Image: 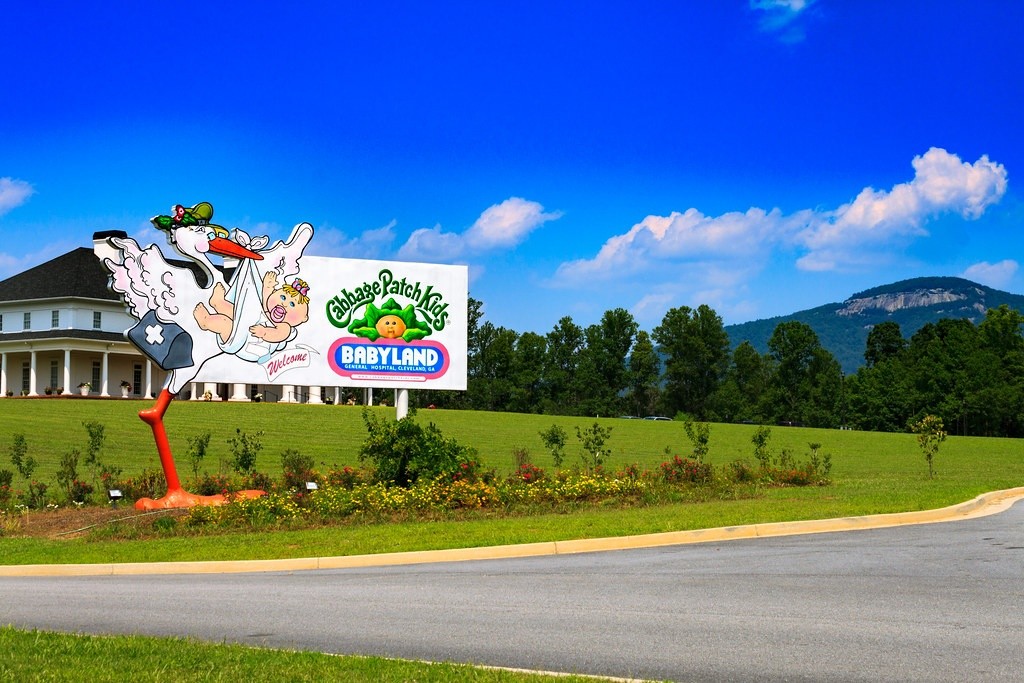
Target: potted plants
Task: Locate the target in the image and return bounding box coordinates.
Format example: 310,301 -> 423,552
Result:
45,386 -> 63,395
119,380 -> 132,398
76,382 -> 93,396
6,390 -> 29,397
